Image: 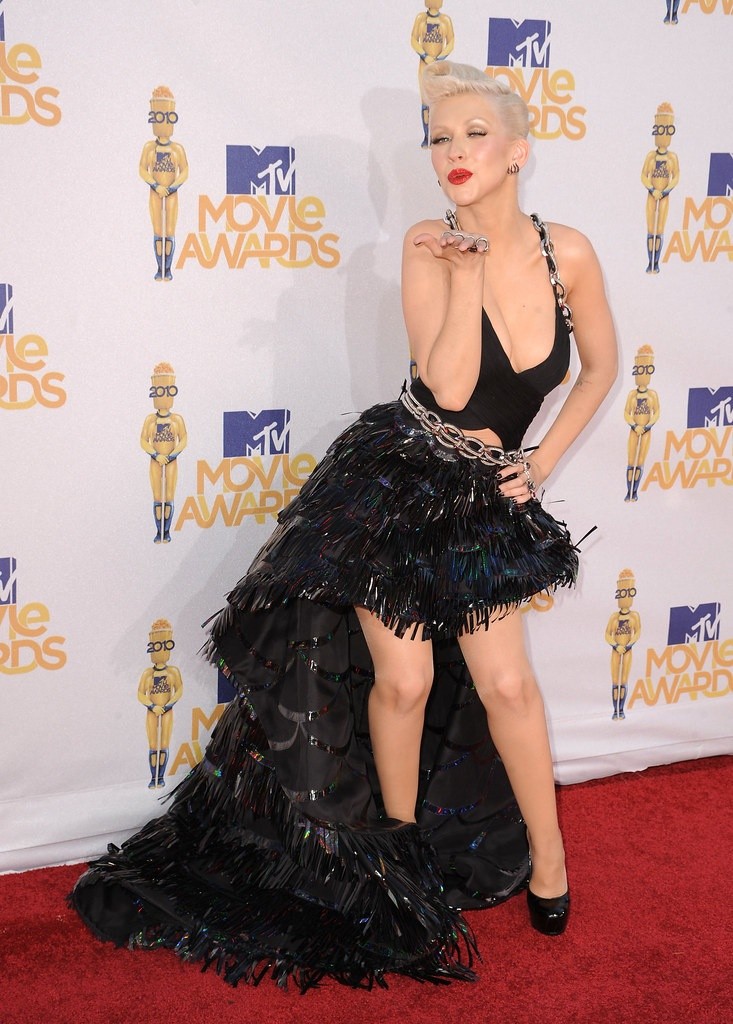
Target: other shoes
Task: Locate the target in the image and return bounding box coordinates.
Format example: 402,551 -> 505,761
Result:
526,846 -> 569,935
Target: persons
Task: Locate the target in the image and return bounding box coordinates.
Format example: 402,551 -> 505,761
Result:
331,60 -> 623,938
